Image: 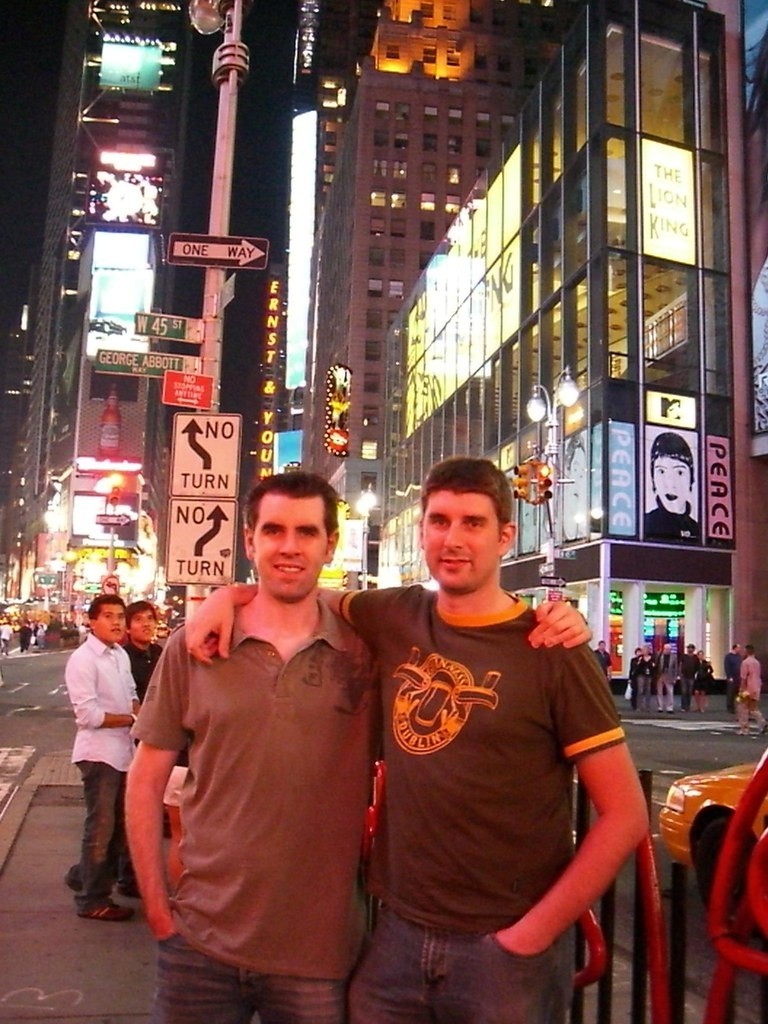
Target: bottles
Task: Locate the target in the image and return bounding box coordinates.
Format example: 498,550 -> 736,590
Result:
98,384 -> 124,458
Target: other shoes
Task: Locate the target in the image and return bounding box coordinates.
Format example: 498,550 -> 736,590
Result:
700,707 -> 704,713
666,707 -> 674,713
657,707 -> 663,712
681,708 -> 685,713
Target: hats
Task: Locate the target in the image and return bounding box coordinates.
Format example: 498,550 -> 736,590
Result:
745,644 -> 754,649
687,645 -> 695,648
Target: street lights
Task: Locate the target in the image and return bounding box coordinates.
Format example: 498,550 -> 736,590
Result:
353,484 -> 376,591
187,0 -> 248,633
525,364 -> 578,598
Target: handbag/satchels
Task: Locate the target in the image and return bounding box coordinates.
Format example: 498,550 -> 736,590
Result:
624,683 -> 633,701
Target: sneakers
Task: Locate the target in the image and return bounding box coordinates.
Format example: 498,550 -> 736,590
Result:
735,728 -> 748,736
117,881 -> 141,898
759,718 -> 768,734
76,897 -> 136,921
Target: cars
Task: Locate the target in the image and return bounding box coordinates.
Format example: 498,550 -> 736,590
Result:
1,616 -> 21,632
158,624 -> 170,638
657,754 -> 768,906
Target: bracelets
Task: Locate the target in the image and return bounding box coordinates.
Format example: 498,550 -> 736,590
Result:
129,713 -> 137,722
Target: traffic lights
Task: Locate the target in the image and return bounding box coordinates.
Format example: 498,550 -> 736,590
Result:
133,312 -> 205,344
538,463 -> 552,503
513,463 -> 529,503
108,476 -> 122,508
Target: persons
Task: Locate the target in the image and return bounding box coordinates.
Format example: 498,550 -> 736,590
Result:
63,594 -> 144,922
186,455 -> 649,1023
694,651 -> 714,712
731,643 -> 768,737
678,644 -> 701,713
631,646 -> 656,714
723,644 -> 742,714
629,648 -> 642,712
0,619 -> 91,656
120,473 -> 591,1023
121,600 -> 165,901
653,644 -> 679,713
592,641 -> 612,684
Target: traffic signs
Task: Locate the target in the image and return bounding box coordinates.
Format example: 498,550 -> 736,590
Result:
96,514 -> 131,526
164,410 -> 241,592
166,232 -> 270,271
91,347 -> 201,385
538,563 -> 556,576
541,578 -> 566,587
